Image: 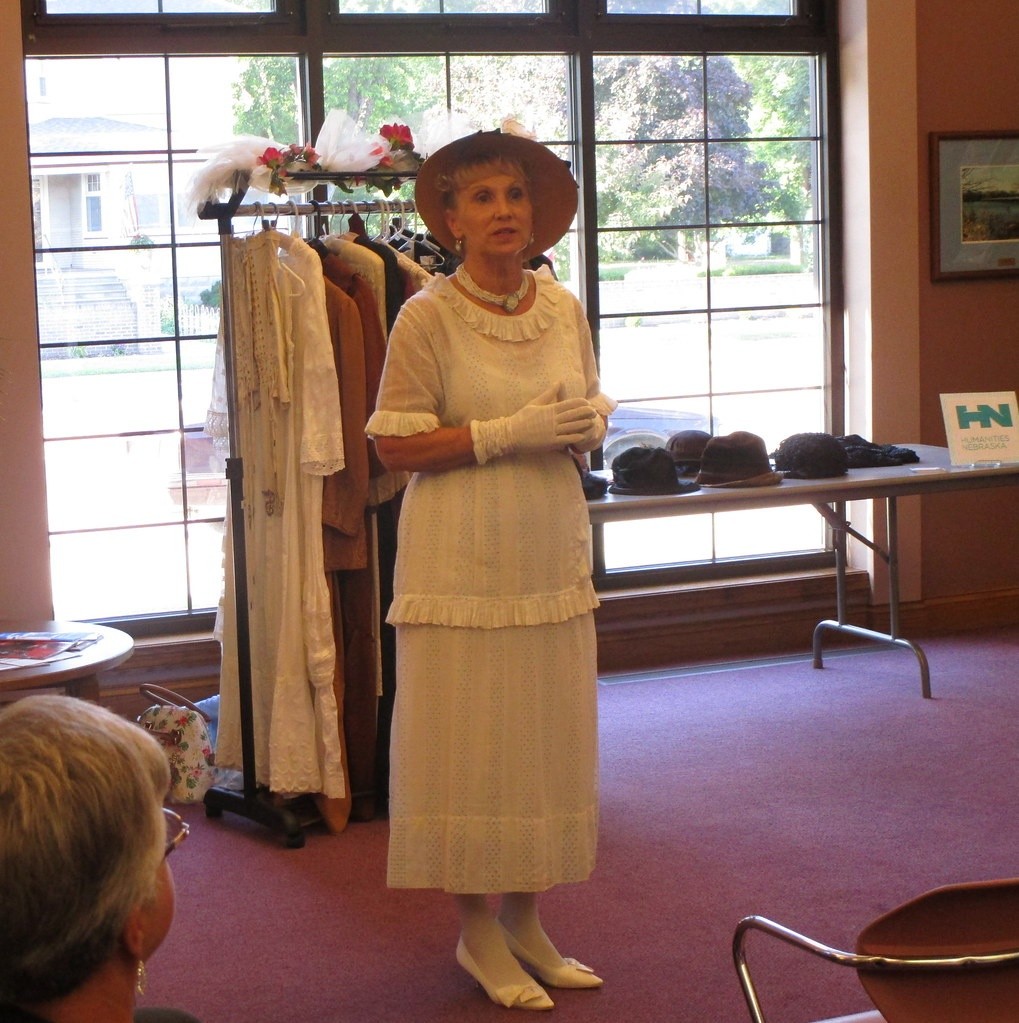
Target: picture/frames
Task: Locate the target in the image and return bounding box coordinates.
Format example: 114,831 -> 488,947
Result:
928,128 -> 1019,281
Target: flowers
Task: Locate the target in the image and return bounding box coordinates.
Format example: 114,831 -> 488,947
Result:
334,124 -> 425,197
259,142 -> 322,196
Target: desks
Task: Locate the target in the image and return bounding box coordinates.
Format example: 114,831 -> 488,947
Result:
581,443 -> 1019,696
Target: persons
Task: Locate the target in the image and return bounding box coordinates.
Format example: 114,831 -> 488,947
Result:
363,130 -> 616,1013
0,692 -> 197,1023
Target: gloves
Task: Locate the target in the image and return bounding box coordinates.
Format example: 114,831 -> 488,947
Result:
470,381 -> 597,465
557,384 -> 607,453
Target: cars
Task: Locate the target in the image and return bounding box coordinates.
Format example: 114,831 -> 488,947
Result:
167,399 -> 711,518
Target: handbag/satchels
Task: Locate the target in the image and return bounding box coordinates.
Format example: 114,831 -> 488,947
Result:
135,682 -> 216,805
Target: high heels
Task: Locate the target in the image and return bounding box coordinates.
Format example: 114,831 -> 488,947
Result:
454,933 -> 554,1011
494,914 -> 604,988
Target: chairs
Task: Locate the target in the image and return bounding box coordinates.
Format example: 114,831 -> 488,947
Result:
732,877 -> 1019,1022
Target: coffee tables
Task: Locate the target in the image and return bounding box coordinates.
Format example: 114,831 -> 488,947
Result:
0,619 -> 133,703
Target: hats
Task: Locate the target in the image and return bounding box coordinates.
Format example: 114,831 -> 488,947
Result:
664,430 -> 716,477
775,432 -> 848,478
569,449 -> 609,501
694,430 -> 784,487
609,445 -> 700,495
415,128 -> 580,261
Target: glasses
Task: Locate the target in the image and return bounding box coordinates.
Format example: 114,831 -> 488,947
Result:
160,806 -> 190,864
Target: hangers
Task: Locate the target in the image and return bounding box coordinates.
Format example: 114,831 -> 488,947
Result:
236,201 -> 446,300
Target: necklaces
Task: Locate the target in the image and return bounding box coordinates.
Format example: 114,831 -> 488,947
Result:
456,262 -> 530,313
